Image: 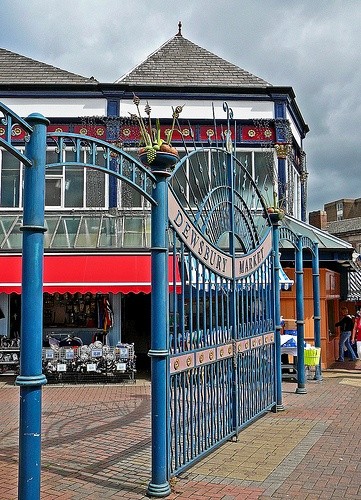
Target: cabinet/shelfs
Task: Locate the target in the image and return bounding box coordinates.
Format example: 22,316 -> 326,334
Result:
0,334 -> 138,385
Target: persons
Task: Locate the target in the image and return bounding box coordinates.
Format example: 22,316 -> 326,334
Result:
335,305 -> 358,362
351,306 -> 361,361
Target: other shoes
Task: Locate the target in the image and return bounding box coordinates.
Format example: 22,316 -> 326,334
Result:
349,360 -> 353,361
335,360 -> 342,362
